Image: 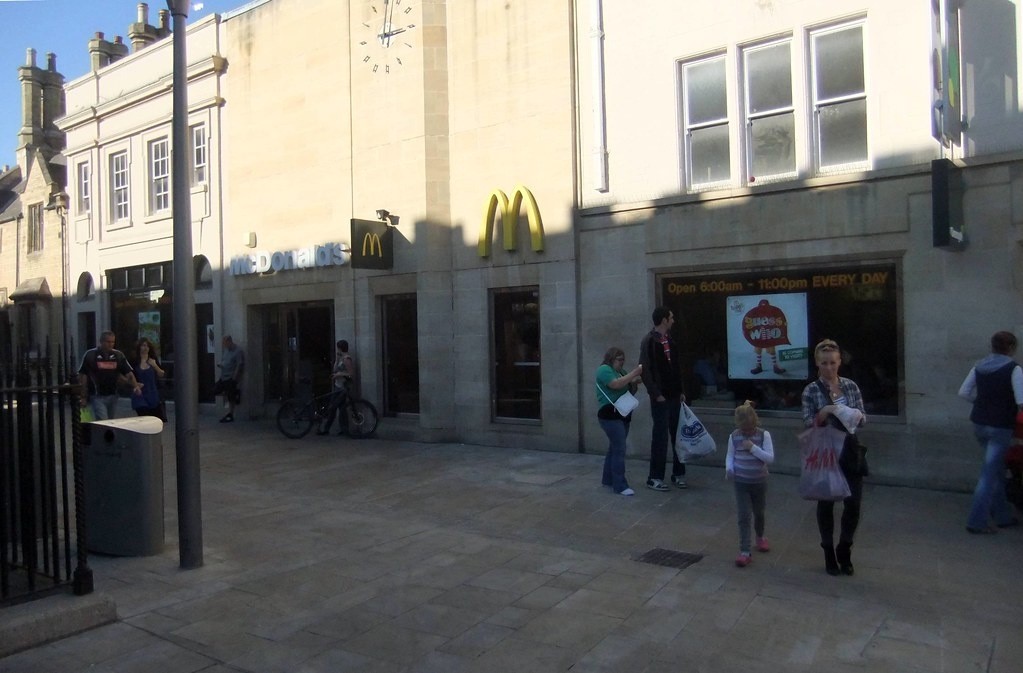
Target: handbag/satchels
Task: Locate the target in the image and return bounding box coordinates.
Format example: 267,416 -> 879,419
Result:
858,445 -> 869,477
155,404 -> 168,422
796,413 -> 852,502
80,405 -> 95,422
674,401 -> 717,464
613,391 -> 639,417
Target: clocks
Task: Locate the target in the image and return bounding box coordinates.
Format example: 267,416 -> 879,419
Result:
358,0 -> 416,73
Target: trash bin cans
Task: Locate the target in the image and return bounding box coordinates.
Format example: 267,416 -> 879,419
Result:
82,415 -> 166,557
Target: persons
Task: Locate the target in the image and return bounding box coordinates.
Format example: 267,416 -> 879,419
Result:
726,401 -> 773,565
959,332 -> 1023,533
78,331 -> 141,420
120,338 -> 165,416
802,340 -> 867,576
639,306 -> 688,490
318,340 -> 353,435
751,299 -> 785,374
213,336 -> 244,422
595,348 -> 643,496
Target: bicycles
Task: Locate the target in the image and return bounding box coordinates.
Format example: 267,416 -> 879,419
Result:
275,377 -> 380,440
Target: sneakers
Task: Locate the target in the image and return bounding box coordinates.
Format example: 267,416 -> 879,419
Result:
735,554 -> 751,566
671,475 -> 688,488
647,479 -> 669,490
755,536 -> 769,551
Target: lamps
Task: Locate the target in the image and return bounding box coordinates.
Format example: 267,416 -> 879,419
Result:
375,209 -> 388,222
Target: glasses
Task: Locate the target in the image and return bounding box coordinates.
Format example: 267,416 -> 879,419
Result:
615,358 -> 625,361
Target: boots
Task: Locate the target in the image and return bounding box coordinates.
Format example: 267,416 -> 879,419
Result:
836,544 -> 854,575
820,542 -> 839,577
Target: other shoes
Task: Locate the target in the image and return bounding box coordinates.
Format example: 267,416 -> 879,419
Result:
620,488 -> 635,495
966,525 -> 998,534
235,389 -> 242,405
219,413 -> 233,423
336,431 -> 344,435
318,431 -> 330,436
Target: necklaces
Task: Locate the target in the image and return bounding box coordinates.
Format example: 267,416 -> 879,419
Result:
831,390 -> 841,397
